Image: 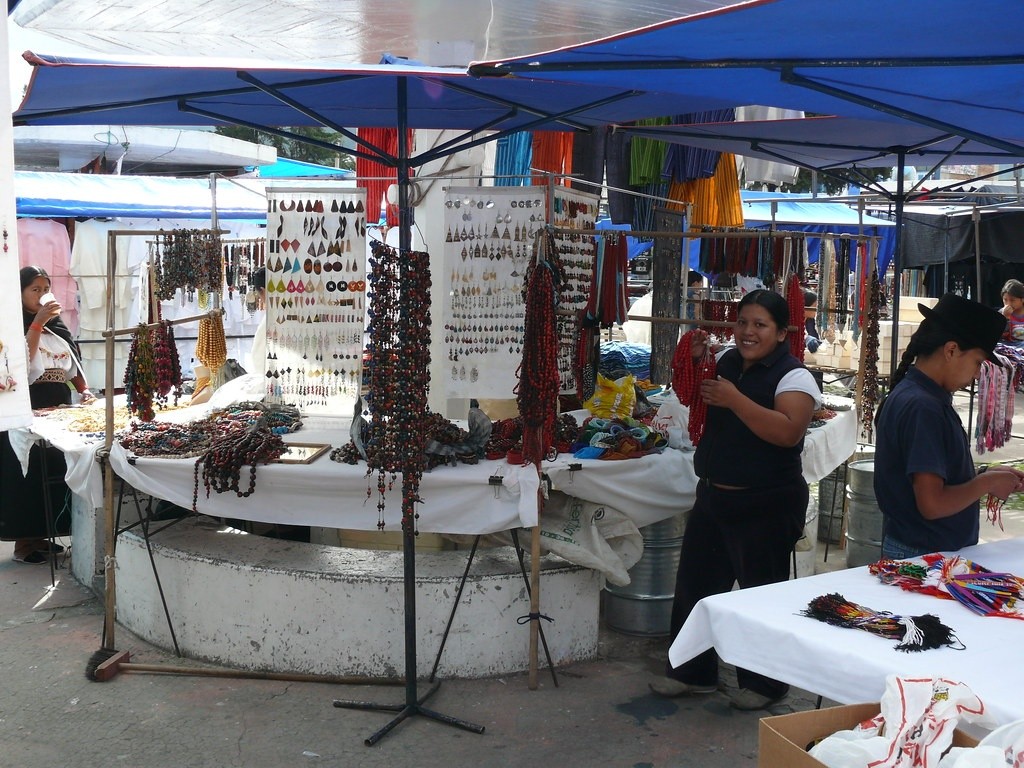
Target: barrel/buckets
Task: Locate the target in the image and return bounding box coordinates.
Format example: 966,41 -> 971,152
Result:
604,511 -> 687,635
845,459 -> 884,569
818,443 -> 875,544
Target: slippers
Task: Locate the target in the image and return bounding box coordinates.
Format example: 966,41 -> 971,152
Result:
11,552 -> 47,565
35,542 -> 63,553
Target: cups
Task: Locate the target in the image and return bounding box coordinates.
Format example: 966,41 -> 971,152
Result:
40,293 -> 61,313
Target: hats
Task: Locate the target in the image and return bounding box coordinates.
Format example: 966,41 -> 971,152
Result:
916,293 -> 1008,367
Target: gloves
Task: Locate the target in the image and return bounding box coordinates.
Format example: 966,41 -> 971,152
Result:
805,335 -> 823,353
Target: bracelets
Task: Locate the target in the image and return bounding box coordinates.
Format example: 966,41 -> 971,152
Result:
28,322 -> 43,333
485,412 -> 667,463
978,465 -> 988,474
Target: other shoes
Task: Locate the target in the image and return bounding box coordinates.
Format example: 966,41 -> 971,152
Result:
649,676 -> 719,696
731,687 -> 790,711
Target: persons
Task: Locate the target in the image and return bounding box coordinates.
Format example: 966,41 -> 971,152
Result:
649,289 -> 821,710
997,279 -> 1024,333
1,266 -> 94,563
874,292 -> 1024,562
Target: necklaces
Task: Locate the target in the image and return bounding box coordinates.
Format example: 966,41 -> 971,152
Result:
580,227 -> 879,449
69,228 -> 571,536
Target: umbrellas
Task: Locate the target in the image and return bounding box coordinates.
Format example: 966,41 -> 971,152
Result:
12,0 -> 1024,370
